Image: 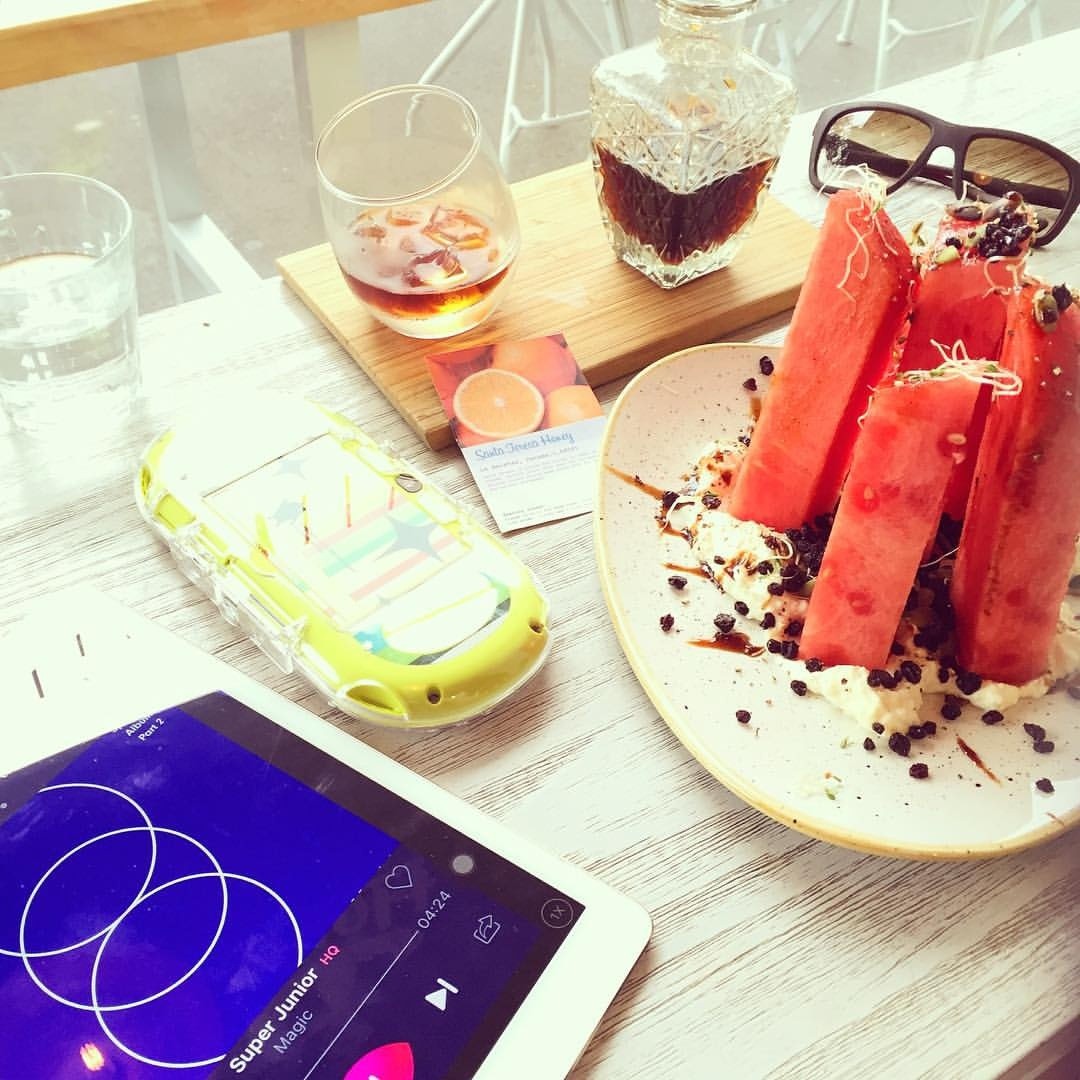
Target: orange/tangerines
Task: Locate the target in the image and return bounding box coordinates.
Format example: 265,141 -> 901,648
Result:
423,336 -> 602,448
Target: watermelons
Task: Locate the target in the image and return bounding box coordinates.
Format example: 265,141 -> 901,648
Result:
725,181 -> 1080,689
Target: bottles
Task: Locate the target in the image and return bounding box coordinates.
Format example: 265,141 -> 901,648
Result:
585,0 -> 798,292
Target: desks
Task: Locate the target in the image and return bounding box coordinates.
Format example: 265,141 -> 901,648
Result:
0,28 -> 1080,1080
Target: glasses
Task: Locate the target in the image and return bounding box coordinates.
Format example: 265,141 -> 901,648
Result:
806,98 -> 1076,249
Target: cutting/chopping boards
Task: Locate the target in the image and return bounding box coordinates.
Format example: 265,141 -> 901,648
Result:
272,153 -> 861,452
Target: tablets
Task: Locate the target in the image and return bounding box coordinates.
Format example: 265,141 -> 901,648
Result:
0,591 -> 653,1080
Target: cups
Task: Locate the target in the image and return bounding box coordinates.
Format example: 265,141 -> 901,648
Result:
0,169 -> 141,438
315,83 -> 518,339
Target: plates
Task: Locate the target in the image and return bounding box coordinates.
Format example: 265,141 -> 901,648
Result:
596,333 -> 1080,856
